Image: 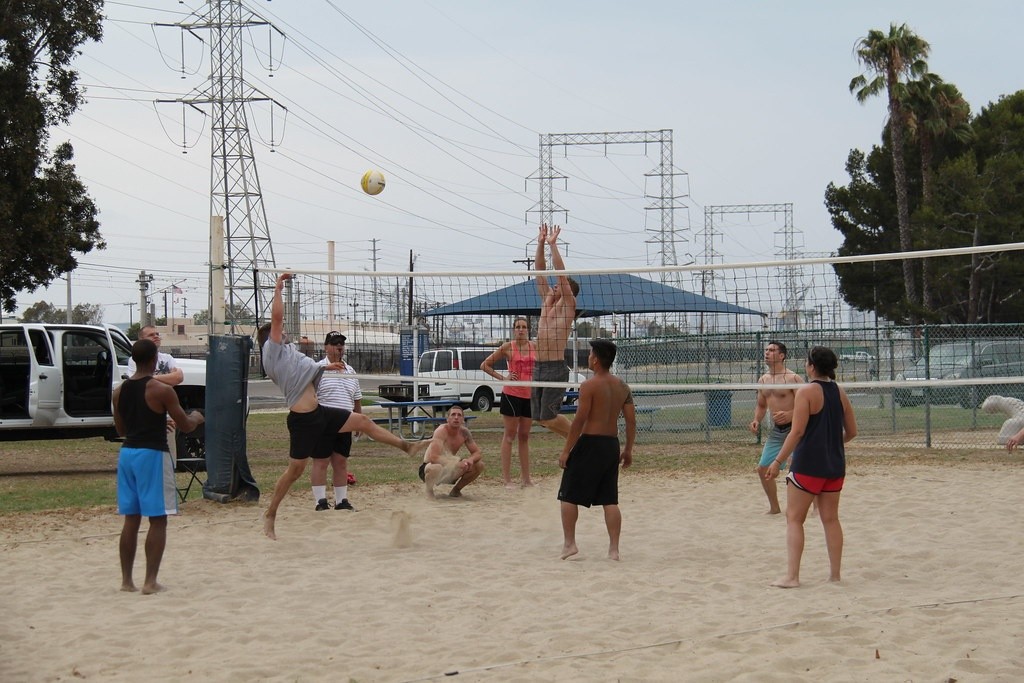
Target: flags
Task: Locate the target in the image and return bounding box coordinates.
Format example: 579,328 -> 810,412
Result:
173,286 -> 182,294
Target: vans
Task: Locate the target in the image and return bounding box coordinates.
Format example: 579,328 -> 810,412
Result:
417,347 -> 587,412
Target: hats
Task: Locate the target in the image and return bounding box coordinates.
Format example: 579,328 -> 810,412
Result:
325,330 -> 347,345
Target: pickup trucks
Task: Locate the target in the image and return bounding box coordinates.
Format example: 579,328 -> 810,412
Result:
839,351 -> 876,364
0,322 -> 251,440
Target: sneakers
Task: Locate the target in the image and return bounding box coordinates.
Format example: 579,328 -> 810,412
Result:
315,497 -> 332,511
335,498 -> 357,512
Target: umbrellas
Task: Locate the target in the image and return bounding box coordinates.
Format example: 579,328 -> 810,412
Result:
414,273 -> 768,392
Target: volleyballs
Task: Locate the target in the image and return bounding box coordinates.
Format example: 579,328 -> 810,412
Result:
361,169 -> 385,195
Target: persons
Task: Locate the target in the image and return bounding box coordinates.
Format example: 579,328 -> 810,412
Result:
112,339 -> 205,594
481,317 -> 536,486
128,325 -> 183,514
258,271 -> 433,540
1006,427 -> 1024,452
310,331 -> 356,511
869,357 -> 876,380
749,342 -> 819,520
530,224 -> 580,437
420,407 -> 485,498
557,340 -> 636,562
764,346 -> 857,587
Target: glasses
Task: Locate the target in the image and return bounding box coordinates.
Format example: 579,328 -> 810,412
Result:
328,340 -> 345,346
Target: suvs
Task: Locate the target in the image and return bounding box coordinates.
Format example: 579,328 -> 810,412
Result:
892,337 -> 1024,407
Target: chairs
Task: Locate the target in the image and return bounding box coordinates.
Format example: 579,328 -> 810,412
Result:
174,429 -> 205,502
0,366 -> 27,411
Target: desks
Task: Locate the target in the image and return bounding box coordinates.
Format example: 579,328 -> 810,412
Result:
372,400 -> 460,441
565,391 -> 579,398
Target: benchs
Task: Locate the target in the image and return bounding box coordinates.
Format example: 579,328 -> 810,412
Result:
371,416 -> 477,422
559,406 -> 662,415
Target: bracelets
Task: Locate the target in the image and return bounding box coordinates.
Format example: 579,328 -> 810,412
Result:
775,458 -> 782,465
